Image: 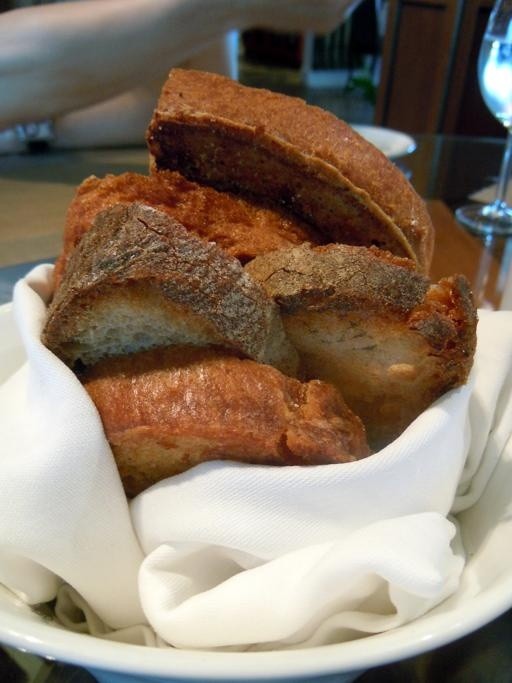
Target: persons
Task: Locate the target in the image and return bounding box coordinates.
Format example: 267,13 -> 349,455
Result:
0,0 -> 362,157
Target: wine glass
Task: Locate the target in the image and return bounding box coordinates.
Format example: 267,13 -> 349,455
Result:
457,0 -> 512,243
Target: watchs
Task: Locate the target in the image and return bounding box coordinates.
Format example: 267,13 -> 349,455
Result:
15,117 -> 57,155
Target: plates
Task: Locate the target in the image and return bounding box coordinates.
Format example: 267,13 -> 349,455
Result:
0,292 -> 509,682
316,122 -> 418,167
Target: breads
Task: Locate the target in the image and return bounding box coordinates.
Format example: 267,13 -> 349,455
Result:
41,65 -> 480,496
